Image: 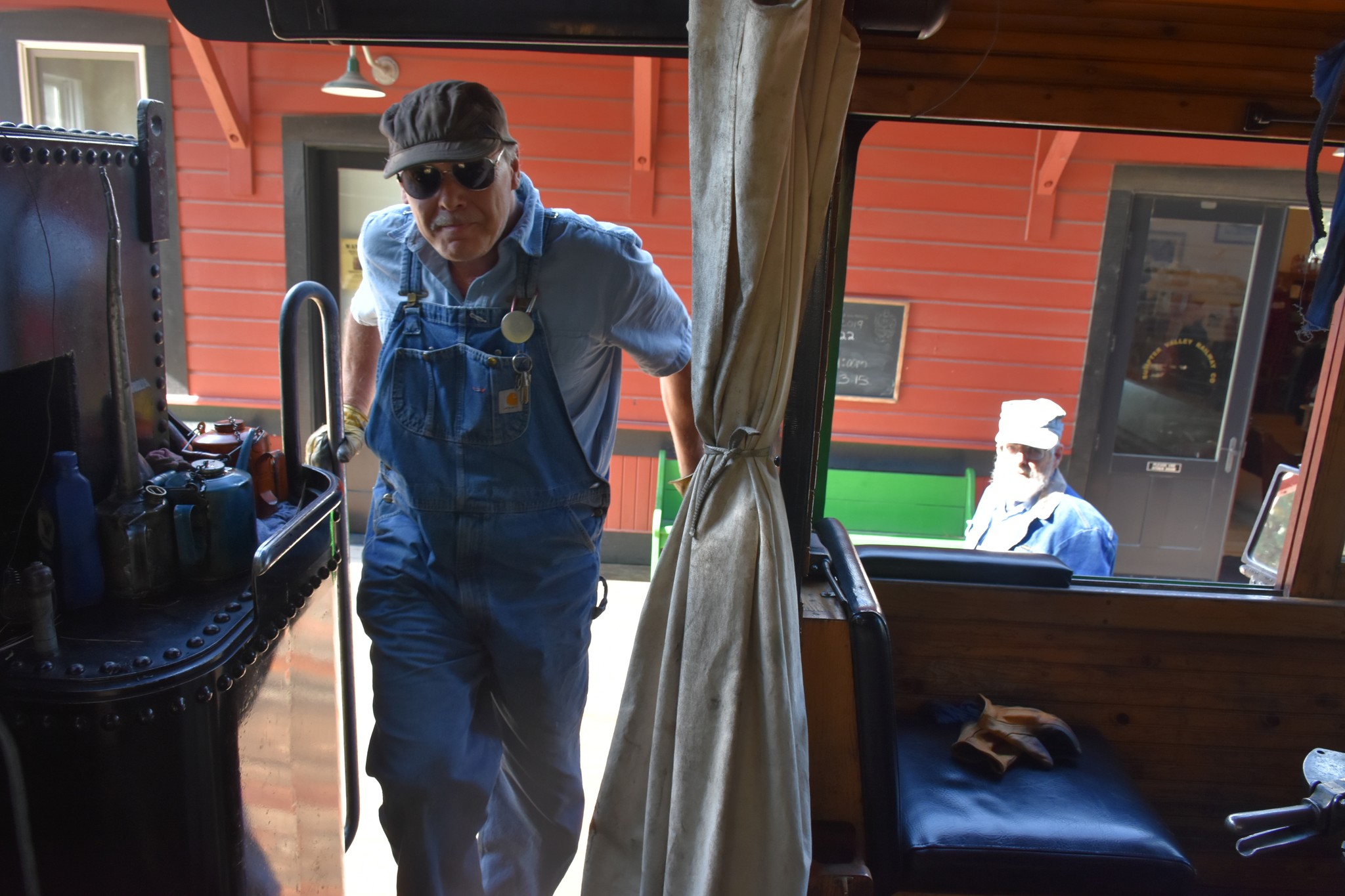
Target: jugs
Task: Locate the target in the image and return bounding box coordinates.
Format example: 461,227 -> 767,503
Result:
106,418 -> 271,607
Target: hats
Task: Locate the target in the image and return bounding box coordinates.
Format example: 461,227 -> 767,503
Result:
995,398 -> 1067,449
378,80 -> 518,178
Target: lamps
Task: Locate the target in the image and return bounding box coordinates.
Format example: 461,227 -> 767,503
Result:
321,44 -> 399,97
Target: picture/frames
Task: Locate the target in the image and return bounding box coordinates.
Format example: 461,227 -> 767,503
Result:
1144,230 -> 1186,268
1214,223 -> 1259,244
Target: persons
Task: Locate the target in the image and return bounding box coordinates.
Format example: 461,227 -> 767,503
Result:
305,80 -> 704,896
965,398 -> 1118,576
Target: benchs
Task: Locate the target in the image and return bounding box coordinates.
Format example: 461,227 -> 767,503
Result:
649,450 -> 976,578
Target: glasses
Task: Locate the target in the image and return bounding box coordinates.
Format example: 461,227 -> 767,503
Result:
396,149 -> 505,199
996,445 -> 1050,460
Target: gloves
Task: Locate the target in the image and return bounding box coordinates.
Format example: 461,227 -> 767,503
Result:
306,404 -> 367,473
977,691 -> 1081,767
953,724 -> 1019,773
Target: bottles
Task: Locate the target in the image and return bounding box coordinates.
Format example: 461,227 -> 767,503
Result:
46,452 -> 105,612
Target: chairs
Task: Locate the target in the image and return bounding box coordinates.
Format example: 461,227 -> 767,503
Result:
810,513 -> 1195,895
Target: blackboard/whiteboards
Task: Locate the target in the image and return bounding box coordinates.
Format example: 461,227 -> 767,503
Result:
835,295 -> 911,405
